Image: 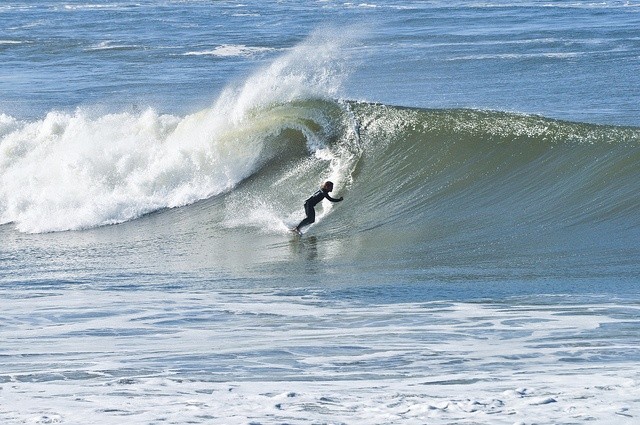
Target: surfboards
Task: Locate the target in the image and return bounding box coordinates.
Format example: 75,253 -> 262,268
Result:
283,222 -> 303,236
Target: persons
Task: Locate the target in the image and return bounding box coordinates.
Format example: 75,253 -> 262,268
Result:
292,181 -> 344,232
